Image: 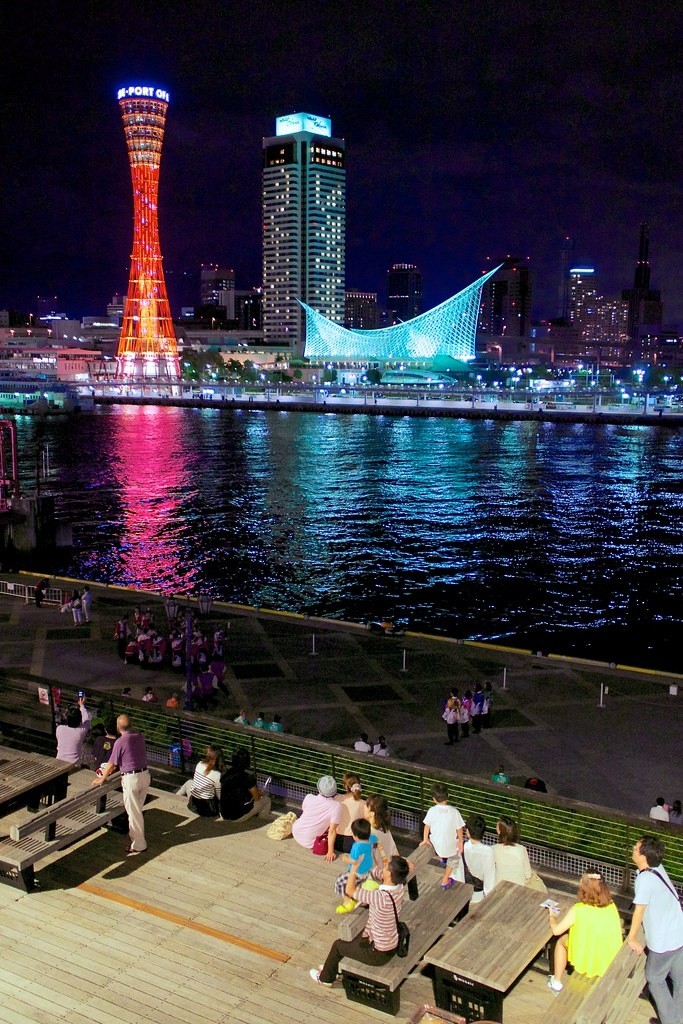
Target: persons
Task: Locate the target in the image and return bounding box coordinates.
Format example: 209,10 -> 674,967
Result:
354,733 -> 390,756
113,606 -> 224,672
335,795 -> 399,914
176,745 -> 271,822
291,775 -> 342,862
548,868 -> 624,997
310,853 -> 409,987
121,687 -> 180,709
649,797 -> 683,825
56,695 -> 119,771
419,784 -> 532,902
71,586 -> 93,626
92,715 -> 151,852
524,778 -> 547,794
627,836 -> 683,1024
492,765 -> 510,784
34,578 -> 50,608
334,773 -> 365,853
442,682 -> 492,745
233,709 -> 283,732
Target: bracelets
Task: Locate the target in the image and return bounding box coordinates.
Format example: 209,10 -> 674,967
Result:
550,915 -> 556,919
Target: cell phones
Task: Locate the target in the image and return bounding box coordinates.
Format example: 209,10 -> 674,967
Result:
78,690 -> 83,700
547,903 -> 558,913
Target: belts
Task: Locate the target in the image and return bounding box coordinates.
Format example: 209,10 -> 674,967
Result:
121,767 -> 148,776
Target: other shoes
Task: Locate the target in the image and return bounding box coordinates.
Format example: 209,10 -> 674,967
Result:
649,1017 -> 662,1024
125,845 -> 147,852
441,878 -> 453,890
547,975 -> 564,996
335,899 -> 355,914
441,858 -> 447,867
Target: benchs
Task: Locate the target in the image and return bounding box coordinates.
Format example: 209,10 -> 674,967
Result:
337,837 -> 474,1016
538,920 -> 648,1024
0,770 -> 127,895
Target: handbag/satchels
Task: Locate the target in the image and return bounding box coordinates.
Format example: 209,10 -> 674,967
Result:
73,599 -> 81,608
461,841 -> 484,892
397,922 -> 410,957
267,812 -> 297,840
42,589 -> 47,594
313,826 -> 329,855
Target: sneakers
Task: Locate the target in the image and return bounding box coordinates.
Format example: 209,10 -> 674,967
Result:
319,964 -> 343,981
309,969 -> 333,987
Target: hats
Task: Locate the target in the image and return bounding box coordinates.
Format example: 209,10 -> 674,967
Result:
317,775 -> 337,797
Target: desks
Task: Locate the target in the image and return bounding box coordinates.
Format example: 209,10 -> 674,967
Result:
0,752 -> 75,820
424,879 -> 579,1024
